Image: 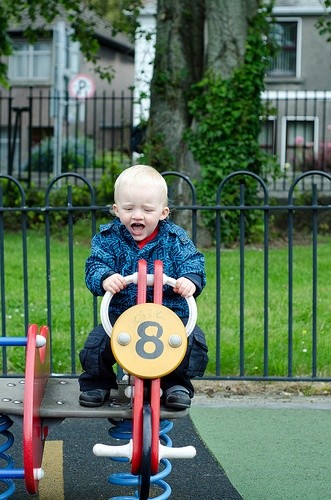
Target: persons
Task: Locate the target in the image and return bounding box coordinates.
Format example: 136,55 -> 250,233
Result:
78,164 -> 209,412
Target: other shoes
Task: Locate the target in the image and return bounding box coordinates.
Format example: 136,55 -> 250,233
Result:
79,389 -> 111,406
166,386 -> 191,408
